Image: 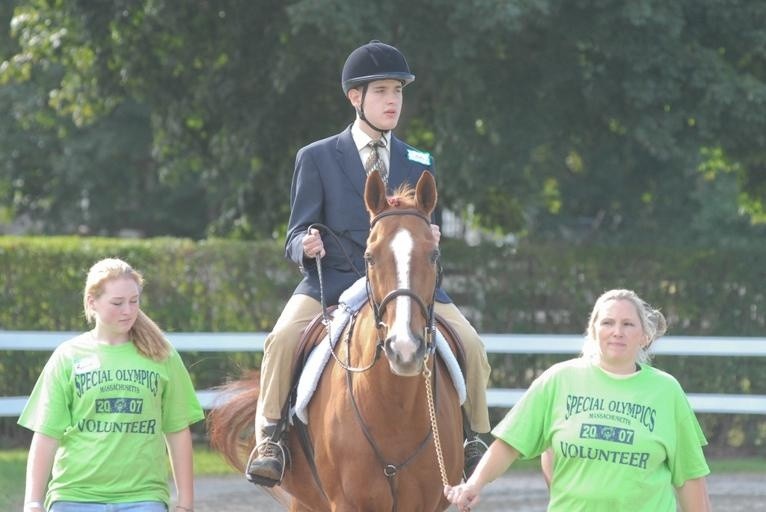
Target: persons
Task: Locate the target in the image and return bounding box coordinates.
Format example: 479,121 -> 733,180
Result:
248,39 -> 492,481
16,257 -> 205,512
442,288 -> 711,512
541,310 -> 667,488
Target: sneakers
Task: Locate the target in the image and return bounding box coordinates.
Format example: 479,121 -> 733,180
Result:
464,432 -> 489,479
248,424 -> 292,482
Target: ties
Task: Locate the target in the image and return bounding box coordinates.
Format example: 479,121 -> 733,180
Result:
365,138 -> 388,182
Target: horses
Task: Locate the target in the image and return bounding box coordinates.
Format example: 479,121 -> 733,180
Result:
205,168 -> 465,512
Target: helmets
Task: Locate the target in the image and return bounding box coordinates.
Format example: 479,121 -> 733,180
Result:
341,39 -> 416,97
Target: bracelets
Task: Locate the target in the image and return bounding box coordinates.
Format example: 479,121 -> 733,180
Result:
176,505 -> 194,512
